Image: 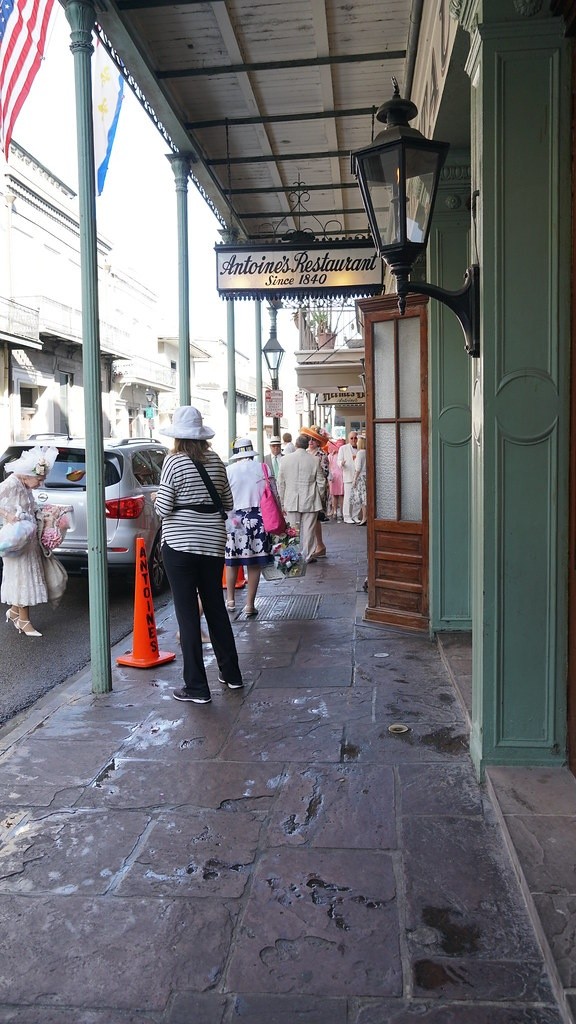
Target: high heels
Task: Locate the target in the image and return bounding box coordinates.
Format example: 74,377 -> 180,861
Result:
16,617 -> 42,636
313,546 -> 327,558
5,608 -> 19,630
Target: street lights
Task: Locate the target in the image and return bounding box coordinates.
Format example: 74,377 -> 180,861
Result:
263,307 -> 285,455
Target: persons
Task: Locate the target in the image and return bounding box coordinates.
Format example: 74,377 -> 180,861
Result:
151,406 -> 244,704
0,445 -> 68,637
320,432 -> 367,526
264,425 -> 329,563
177,587 -> 212,643
223,438 -> 282,615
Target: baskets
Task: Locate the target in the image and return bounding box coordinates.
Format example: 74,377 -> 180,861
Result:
262,530 -> 307,582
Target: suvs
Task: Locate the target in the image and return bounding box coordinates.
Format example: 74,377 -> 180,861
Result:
0,432 -> 174,596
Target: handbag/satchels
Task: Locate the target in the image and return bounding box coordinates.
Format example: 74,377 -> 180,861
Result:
0,508 -> 38,557
261,462 -> 287,534
36,513 -> 68,610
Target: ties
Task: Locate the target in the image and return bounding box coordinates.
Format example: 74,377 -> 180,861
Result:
274,456 -> 279,481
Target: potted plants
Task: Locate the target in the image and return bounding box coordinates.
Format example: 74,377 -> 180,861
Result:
309,310 -> 337,349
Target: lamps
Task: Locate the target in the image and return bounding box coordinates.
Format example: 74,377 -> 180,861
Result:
348,79 -> 479,360
359,357 -> 365,391
337,385 -> 347,391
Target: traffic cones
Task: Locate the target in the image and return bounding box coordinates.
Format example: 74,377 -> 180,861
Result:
116,538 -> 177,668
221,562 -> 249,589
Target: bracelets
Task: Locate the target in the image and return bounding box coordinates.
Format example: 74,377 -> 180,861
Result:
5,511 -> 7,520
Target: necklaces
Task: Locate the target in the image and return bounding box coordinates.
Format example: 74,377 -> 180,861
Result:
22,477 -> 32,514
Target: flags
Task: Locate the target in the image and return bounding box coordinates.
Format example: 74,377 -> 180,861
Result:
96,22 -> 124,196
0,0 -> 55,162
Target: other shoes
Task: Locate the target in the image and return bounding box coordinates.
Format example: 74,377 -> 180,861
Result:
199,634 -> 212,642
327,509 -> 368,526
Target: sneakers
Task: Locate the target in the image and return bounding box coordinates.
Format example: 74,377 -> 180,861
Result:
172,688 -> 212,704
218,670 -> 244,689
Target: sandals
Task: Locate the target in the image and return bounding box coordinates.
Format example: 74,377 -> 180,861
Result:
246,605 -> 258,616
225,599 -> 236,612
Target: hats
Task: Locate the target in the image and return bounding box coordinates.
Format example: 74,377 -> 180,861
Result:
228,438 -> 260,459
268,436 -> 283,446
159,407 -> 215,439
301,425 -> 330,446
